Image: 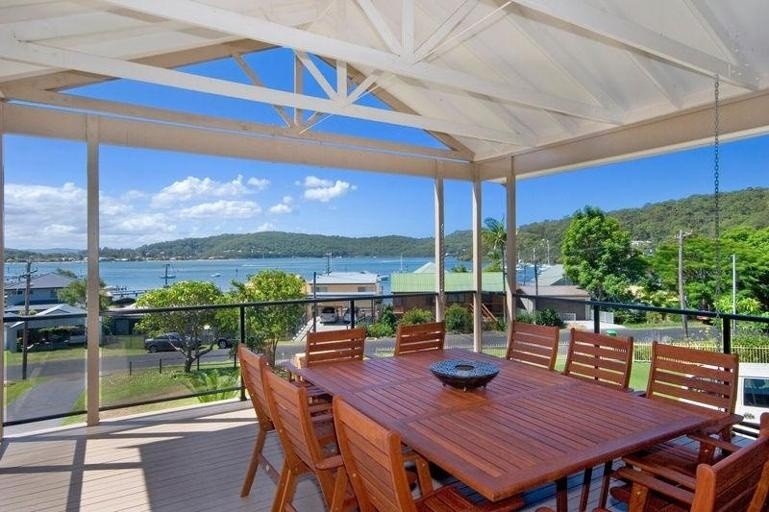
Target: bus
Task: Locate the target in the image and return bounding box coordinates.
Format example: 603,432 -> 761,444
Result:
69,325 -> 112,345
678,361 -> 769,426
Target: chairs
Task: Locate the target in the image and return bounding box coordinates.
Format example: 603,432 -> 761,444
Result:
262,362 -> 418,512
506,323 -> 559,371
395,322 -> 445,355
304,326 -> 365,367
564,328 -> 634,388
332,393 -> 524,512
237,343 -> 331,512
610,340 -> 744,502
591,412 -> 768,512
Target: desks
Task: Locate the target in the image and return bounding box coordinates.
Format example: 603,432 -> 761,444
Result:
295,347 -> 712,512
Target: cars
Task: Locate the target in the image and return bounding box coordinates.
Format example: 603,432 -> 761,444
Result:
219,336 -> 263,348
344,307 -> 365,322
144,332 -> 201,351
320,306 -> 338,324
